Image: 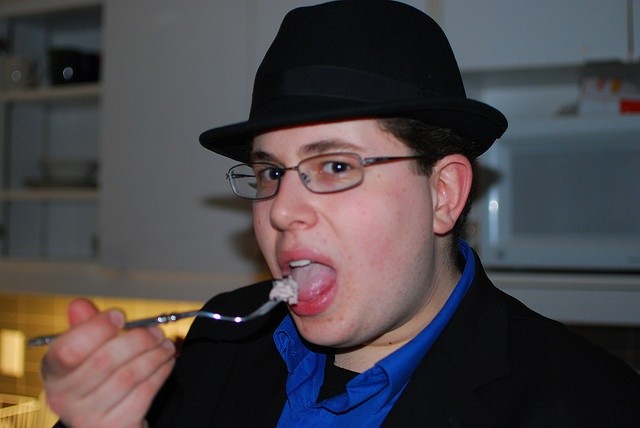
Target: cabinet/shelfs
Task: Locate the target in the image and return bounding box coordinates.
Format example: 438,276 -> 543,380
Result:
0,0 -> 104,266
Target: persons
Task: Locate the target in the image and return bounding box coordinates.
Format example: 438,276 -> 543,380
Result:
40,1 -> 640,428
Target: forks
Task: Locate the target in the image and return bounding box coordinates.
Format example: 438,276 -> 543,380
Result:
26,294 -> 287,347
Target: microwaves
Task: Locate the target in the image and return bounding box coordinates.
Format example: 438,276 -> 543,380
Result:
460,116 -> 640,273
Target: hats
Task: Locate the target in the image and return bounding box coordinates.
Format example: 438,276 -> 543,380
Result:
199,0 -> 508,167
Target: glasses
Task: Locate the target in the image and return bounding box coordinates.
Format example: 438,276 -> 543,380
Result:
225,152 -> 439,200
